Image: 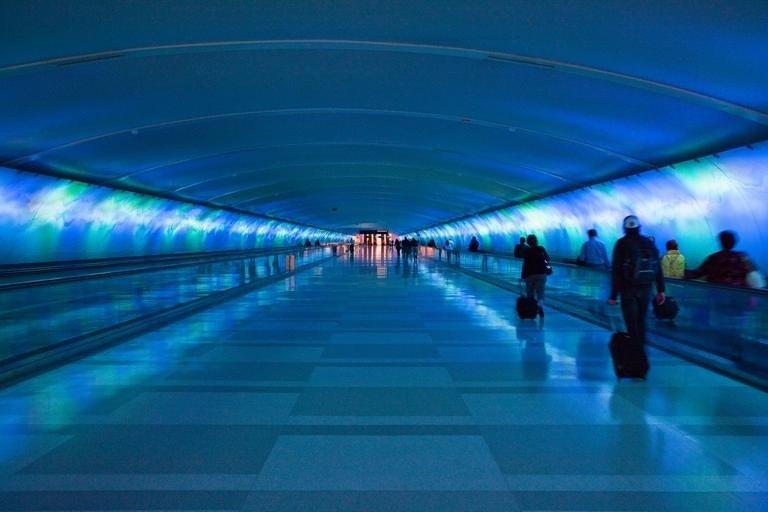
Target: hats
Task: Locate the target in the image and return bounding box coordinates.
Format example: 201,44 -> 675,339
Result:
623,215 -> 641,229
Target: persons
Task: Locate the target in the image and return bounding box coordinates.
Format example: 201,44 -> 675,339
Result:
395,237 -> 453,259
660,239 -> 686,280
578,230 -> 609,271
609,214 -> 666,338
514,238 -> 527,258
470,236 -> 479,252
686,231 -> 755,288
350,238 -> 354,253
522,235 -> 549,318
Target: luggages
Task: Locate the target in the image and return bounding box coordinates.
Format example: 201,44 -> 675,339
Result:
517,279 -> 538,319
652,292 -> 678,319
607,302 -> 648,379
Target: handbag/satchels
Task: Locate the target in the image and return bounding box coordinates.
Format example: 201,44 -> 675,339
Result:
544,259 -> 553,275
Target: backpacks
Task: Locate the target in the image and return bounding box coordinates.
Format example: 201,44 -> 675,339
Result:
625,238 -> 661,286
